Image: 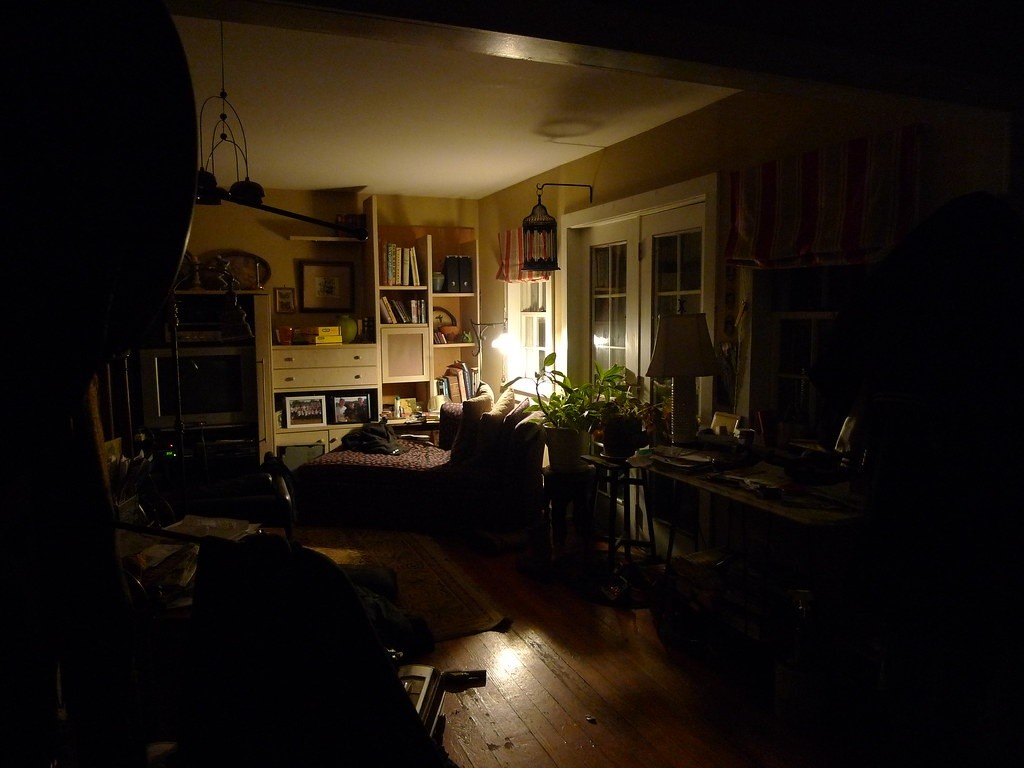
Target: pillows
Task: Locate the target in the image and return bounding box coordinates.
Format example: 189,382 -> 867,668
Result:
449,381 -> 495,459
471,386 -> 514,458
498,397 -> 530,455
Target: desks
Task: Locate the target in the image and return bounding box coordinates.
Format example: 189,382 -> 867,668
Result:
577,453 -> 659,584
629,442 -> 881,694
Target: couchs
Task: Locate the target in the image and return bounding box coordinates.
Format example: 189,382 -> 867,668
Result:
301,381 -> 548,533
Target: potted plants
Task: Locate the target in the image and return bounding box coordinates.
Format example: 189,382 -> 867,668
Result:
500,352 -> 672,457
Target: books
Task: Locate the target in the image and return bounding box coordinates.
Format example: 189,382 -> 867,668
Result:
434,359 -> 479,404
380,239 -> 421,287
380,295 -> 426,325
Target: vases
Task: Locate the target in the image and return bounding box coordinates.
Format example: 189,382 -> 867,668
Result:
432,272 -> 445,292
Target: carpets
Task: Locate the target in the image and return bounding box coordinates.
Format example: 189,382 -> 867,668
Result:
289,527 -> 509,641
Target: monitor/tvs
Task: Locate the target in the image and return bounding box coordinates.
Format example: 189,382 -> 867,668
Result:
139,346 -> 258,429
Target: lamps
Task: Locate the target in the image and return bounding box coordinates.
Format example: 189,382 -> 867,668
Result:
468,318 -> 518,381
647,300 -> 724,447
520,182 -> 592,272
194,19 -> 266,207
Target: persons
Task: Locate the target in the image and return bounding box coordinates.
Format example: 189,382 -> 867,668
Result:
290,400 -> 321,420
336,397 -> 369,423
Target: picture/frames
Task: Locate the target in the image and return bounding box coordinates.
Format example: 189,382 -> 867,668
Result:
285,396 -> 327,428
216,252 -> 271,290
331,395 -> 372,424
297,260 -> 355,313
275,287 -> 295,313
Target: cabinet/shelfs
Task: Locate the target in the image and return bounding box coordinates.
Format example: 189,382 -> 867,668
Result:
271,196 -> 483,471
